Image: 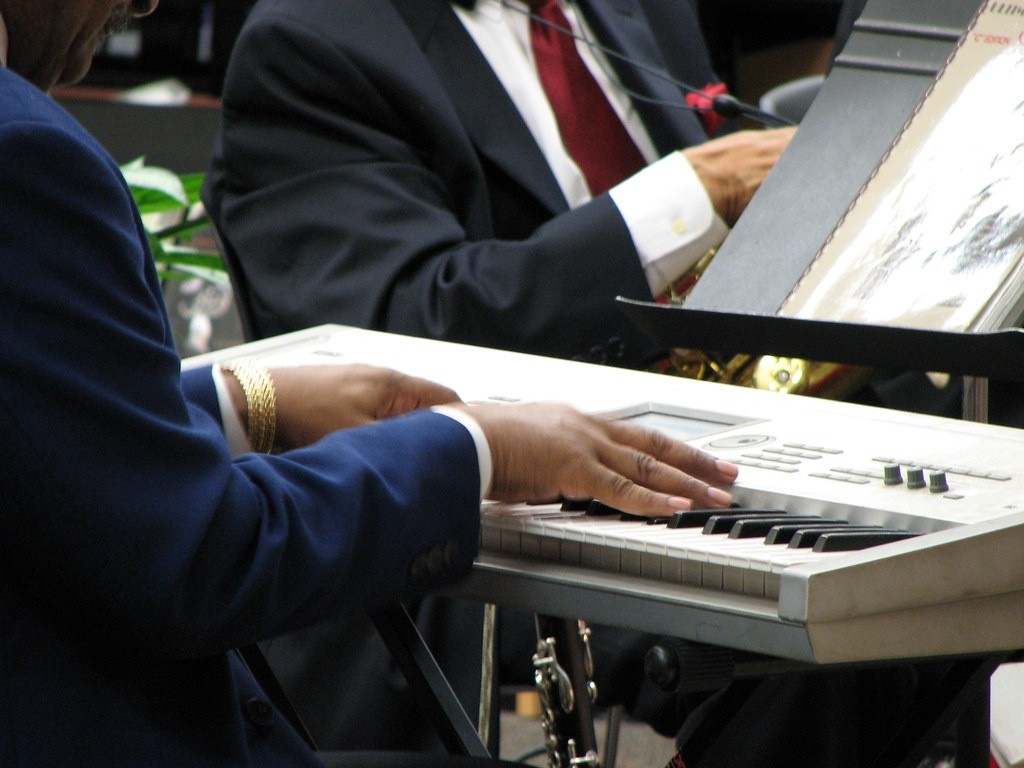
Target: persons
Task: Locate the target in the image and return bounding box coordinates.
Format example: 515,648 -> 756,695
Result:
206,2 -> 988,765
0,4 -> 743,768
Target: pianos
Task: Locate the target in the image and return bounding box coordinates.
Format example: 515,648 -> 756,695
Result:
179,320 -> 1024,670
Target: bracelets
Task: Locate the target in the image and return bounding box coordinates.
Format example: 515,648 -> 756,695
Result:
219,356 -> 278,456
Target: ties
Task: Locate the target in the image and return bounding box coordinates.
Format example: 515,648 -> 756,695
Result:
525,0 -> 701,306
